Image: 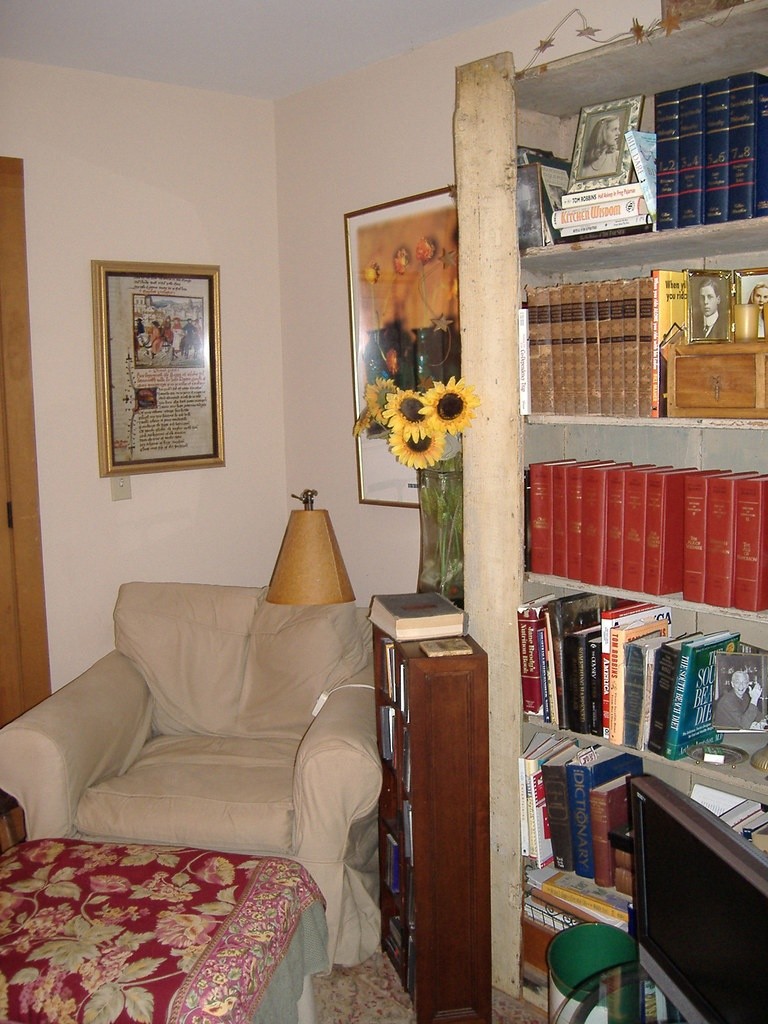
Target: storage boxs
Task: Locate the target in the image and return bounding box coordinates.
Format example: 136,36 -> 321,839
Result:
666,341 -> 768,418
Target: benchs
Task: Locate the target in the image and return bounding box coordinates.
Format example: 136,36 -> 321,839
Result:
0,838 -> 328,1024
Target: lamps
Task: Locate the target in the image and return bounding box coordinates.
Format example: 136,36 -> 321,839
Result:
266,489 -> 356,605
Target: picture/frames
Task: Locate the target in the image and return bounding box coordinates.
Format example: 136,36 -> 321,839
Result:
734,267 -> 768,341
685,270 -> 734,343
91,259 -> 226,478
565,93 -> 644,194
344,185 -> 462,508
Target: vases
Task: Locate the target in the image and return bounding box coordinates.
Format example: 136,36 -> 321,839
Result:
418,469 -> 464,610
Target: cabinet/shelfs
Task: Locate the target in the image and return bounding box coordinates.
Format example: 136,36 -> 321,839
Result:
372,625 -> 492,1024
455,0 -> 768,1024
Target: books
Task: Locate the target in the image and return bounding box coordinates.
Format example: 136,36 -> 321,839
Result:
518,72 -> 768,245
518,731 -> 768,933
381,638 -> 413,992
522,894 -> 582,934
524,458 -> 768,611
517,593 -> 742,762
368,592 -> 469,640
518,271 -> 688,418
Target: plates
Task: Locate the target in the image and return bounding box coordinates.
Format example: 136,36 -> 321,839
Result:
685,744 -> 748,766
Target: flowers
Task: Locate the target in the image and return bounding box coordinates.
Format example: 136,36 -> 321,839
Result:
353,376 -> 481,597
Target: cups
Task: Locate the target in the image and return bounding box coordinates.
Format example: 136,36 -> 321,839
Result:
734,304 -> 759,343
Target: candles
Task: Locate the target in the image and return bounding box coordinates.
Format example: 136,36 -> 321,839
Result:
733,303 -> 759,341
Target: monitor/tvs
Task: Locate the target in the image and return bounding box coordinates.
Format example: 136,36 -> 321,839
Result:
626,773 -> 768,1024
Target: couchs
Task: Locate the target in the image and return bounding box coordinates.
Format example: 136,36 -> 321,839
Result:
0,582 -> 383,976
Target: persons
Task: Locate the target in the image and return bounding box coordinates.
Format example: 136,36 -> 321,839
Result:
581,116 -> 619,174
694,279 -> 724,338
715,671 -> 763,729
748,284 -> 768,337
